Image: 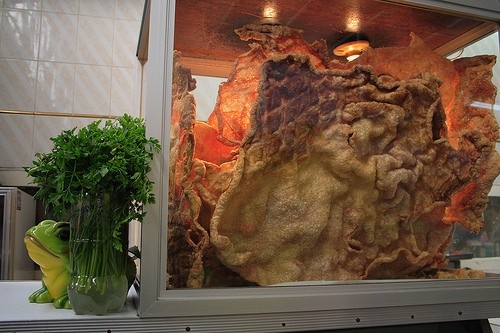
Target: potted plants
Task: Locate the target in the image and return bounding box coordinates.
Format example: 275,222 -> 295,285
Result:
22,113 -> 163,316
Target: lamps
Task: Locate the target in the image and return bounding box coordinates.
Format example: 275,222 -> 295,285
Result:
333,40 -> 370,61
